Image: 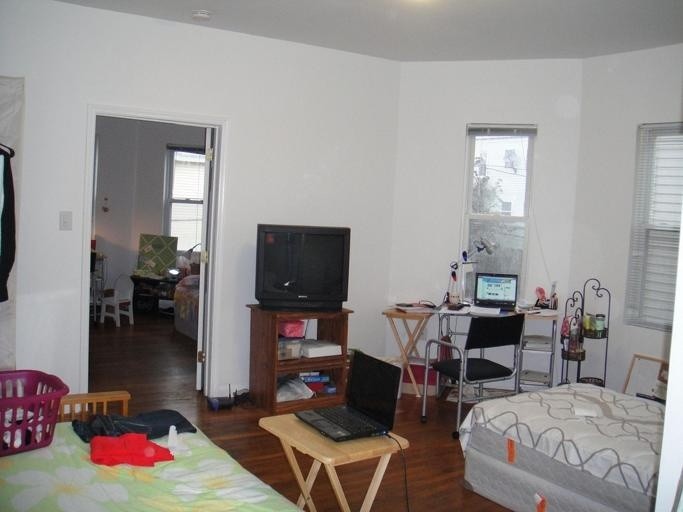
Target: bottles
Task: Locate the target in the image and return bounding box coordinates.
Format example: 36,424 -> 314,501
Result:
165,424 -> 179,454
448,271 -> 460,304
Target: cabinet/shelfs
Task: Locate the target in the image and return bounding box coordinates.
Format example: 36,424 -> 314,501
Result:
246,303 -> 354,415
557,278 -> 612,388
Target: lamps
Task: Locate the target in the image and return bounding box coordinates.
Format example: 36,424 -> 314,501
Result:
443,237 -> 497,305
183,243 -> 201,259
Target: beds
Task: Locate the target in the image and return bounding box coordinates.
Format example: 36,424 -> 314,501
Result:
0,391 -> 306,512
172,275 -> 199,343
459,382 -> 665,512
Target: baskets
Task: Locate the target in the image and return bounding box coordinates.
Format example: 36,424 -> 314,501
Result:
0,367 -> 71,459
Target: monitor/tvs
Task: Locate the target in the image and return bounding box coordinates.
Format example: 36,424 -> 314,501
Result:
255,224 -> 350,309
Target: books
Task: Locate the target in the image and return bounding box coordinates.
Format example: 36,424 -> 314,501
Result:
300,374 -> 329,382
298,371 -> 320,376
394,304 -> 500,316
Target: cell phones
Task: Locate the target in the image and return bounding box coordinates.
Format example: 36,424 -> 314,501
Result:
458,302 -> 470,306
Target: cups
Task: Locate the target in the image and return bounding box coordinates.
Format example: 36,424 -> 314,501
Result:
593,314 -> 605,338
582,312 -> 594,337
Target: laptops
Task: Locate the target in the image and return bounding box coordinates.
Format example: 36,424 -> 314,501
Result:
295,350 -> 401,442
474,273 -> 517,311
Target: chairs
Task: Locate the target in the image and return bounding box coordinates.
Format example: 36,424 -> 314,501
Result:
100,274 -> 134,327
421,312 -> 525,439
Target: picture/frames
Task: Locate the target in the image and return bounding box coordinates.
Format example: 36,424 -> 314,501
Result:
623,353 -> 669,405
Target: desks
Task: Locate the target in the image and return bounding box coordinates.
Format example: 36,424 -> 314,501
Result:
258,414 -> 409,512
435,307 -> 558,401
130,275 -> 182,319
381,308 -> 434,397
